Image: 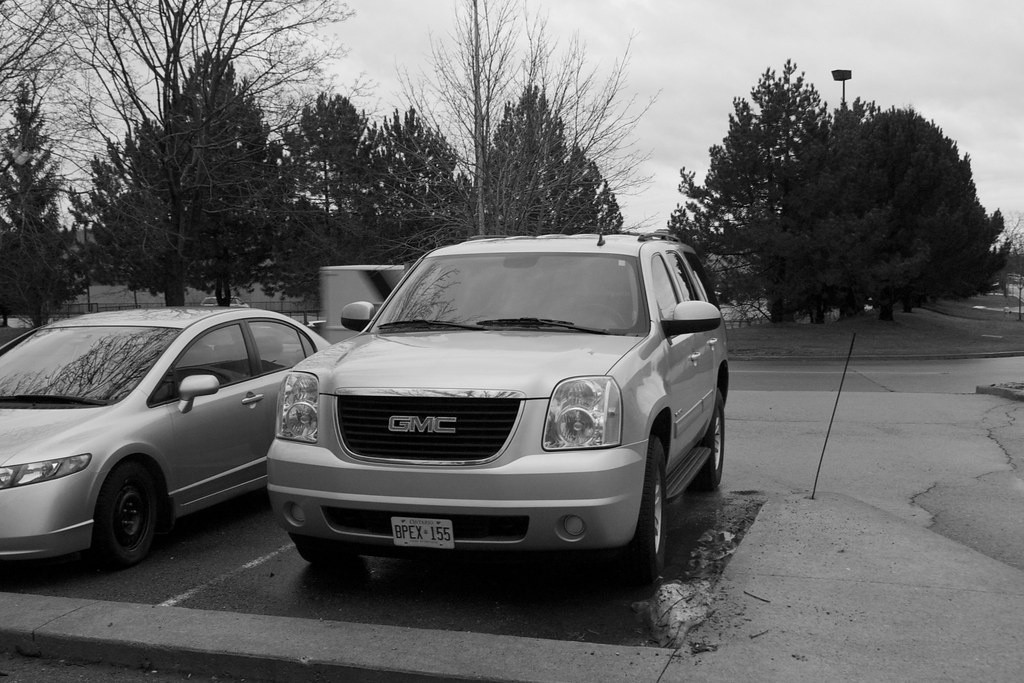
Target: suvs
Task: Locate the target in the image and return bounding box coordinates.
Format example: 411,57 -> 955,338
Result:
266,230 -> 729,590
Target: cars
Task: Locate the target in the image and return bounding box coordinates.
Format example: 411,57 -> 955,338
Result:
201,296 -> 249,308
1,304 -> 331,572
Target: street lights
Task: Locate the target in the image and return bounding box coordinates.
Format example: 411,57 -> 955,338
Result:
833,70 -> 853,107
